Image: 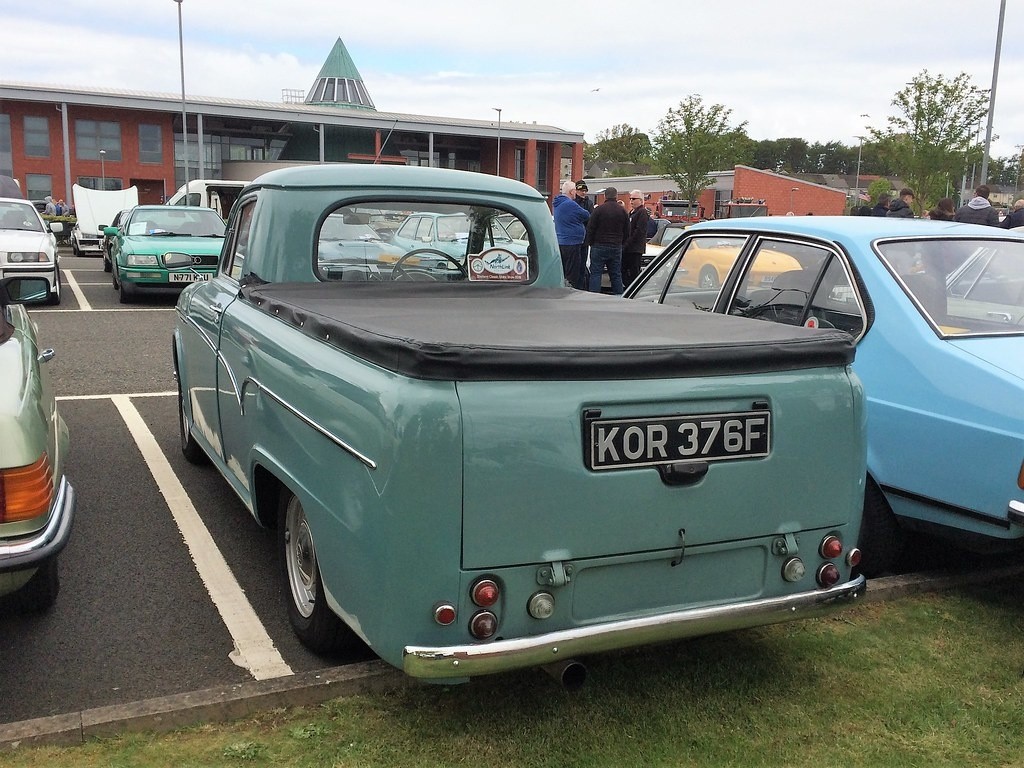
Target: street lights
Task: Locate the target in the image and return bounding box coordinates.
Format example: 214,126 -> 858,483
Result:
491,107 -> 502,176
790,188 -> 800,216
852,136 -> 864,196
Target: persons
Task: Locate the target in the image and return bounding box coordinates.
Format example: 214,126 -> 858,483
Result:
42,197 -> 70,217
786,185 -> 1024,230
552,180 -> 660,296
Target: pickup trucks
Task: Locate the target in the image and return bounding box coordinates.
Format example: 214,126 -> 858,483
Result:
171,165 -> 869,694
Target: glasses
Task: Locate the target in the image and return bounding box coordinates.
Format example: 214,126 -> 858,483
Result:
630,198 -> 640,200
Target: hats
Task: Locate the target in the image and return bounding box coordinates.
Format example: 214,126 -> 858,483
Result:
654,211 -> 661,219
575,180 -> 588,192
646,207 -> 652,216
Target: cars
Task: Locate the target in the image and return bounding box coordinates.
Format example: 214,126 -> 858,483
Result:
506,218 -> 687,289
318,213 -> 531,283
622,215 -> 1024,565
71,179 -> 254,303
0,303 -> 73,613
0,175 -> 63,306
